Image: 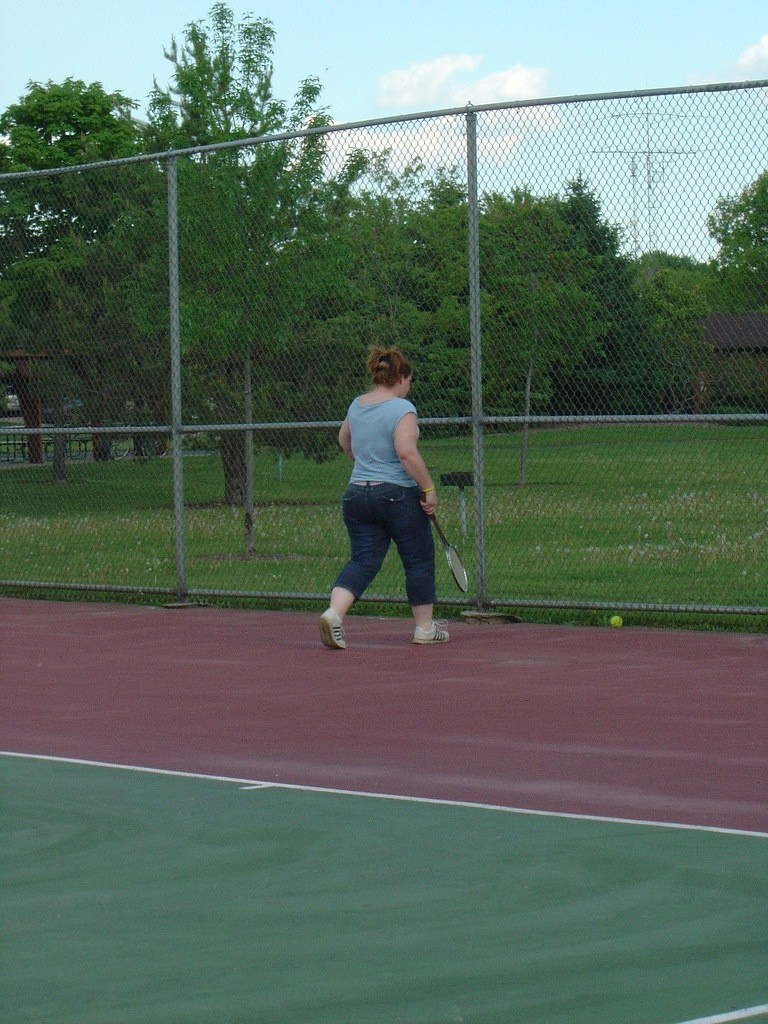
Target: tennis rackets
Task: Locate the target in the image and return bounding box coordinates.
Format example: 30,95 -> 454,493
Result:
418,490 -> 469,592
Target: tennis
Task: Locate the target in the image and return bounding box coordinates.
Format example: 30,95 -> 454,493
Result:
610,616 -> 623,628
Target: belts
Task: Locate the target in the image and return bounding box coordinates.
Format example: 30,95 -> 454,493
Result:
351,479 -> 383,486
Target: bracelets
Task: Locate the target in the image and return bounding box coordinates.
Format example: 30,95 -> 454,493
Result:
421,485 -> 437,493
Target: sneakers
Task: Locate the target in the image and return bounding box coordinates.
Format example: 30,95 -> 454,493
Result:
319,609 -> 347,649
413,619 -> 450,643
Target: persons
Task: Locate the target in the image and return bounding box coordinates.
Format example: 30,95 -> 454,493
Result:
317,343 -> 451,649
